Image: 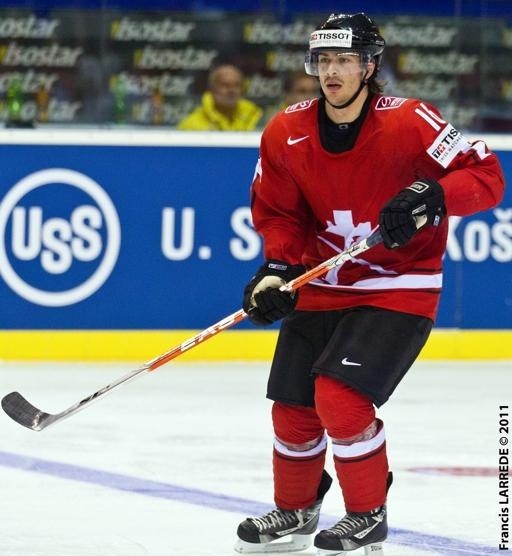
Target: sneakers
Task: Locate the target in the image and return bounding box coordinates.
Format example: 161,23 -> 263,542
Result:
312,470 -> 394,551
236,468 -> 333,544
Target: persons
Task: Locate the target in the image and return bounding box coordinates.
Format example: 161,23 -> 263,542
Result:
237,11 -> 505,552
177,63 -> 265,132
266,70 -> 317,126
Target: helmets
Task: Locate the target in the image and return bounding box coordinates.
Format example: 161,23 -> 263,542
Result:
303,9 -> 387,84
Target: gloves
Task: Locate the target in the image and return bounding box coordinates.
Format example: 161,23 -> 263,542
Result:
375,173 -> 451,253
241,255 -> 308,329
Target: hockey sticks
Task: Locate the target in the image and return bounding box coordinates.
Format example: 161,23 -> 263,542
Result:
1,195 -> 439,432
